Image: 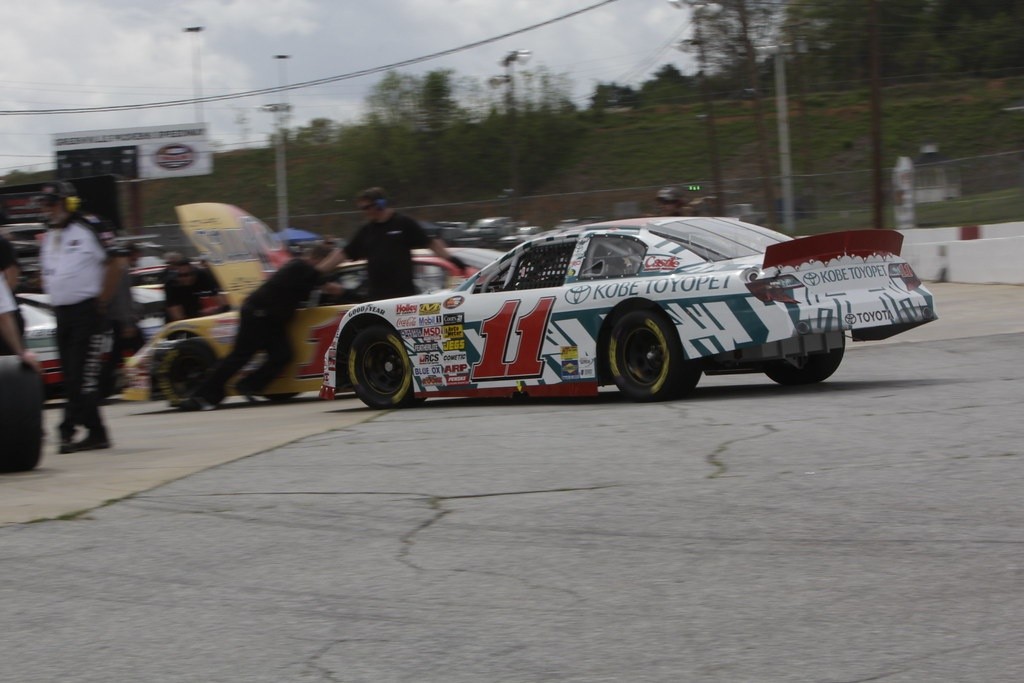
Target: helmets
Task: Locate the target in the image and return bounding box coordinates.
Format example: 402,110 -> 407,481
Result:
355,187 -> 389,211
36,182 -> 72,202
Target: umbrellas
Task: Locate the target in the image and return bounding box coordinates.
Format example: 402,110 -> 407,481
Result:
271,228 -> 320,242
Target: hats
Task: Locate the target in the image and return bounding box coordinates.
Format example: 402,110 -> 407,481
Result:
656,187 -> 684,201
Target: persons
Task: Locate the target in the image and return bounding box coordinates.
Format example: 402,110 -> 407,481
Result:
319,187 -> 477,302
34,181 -> 132,456
185,235 -> 346,410
0,235 -> 44,373
163,251 -> 221,322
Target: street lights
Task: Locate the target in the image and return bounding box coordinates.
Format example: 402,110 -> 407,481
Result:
656,184 -> 689,204
500,47 -> 534,117
182,23 -> 207,134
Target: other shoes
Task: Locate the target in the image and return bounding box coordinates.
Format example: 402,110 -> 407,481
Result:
54,421 -> 110,455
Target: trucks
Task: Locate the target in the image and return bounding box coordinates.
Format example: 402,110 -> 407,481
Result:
0,172 -> 137,258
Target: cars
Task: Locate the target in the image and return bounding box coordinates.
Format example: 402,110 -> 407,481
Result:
317,216 -> 939,419
0,203 -> 585,411
